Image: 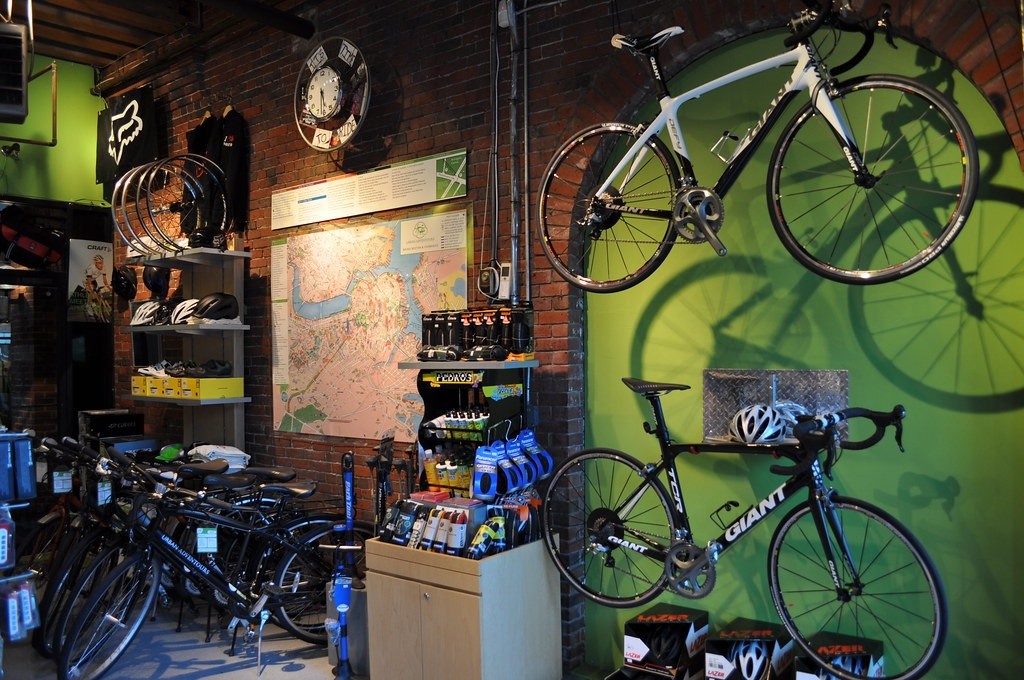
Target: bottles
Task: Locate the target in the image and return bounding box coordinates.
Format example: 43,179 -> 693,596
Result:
423,443 -> 479,500
444,405 -> 490,441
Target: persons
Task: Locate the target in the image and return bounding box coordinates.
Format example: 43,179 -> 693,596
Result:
81,255 -> 112,305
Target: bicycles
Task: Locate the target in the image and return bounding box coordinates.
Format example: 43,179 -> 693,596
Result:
0,424 -> 377,680
541,377 -> 949,680
537,1 -> 982,292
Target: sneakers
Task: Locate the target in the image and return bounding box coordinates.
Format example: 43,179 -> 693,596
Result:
137,359 -> 174,377
165,360 -> 200,378
151,369 -> 175,379
184,360 -> 233,378
85,304 -> 94,316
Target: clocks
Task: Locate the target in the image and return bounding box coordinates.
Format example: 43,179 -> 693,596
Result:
306,65 -> 344,123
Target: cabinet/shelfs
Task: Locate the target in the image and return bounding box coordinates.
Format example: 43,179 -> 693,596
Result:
365,360 -> 564,680
120,235 -> 251,453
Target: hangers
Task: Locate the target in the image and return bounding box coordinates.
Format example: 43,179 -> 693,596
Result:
200,101 -> 211,127
221,94 -> 233,118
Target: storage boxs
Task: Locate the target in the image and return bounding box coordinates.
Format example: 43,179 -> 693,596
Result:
623,602 -> 884,680
77,409 -> 157,455
410,491 -> 487,544
130,375 -> 244,400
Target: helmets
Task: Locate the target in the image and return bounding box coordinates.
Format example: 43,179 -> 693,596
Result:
171,299 -> 200,325
770,400 -> 813,438
191,291 -> 240,320
728,403 -> 786,444
128,301 -> 160,326
186,440 -> 210,461
143,267 -> 169,298
149,301 -> 180,326
643,622 -> 685,667
815,654 -> 863,680
723,637 -> 769,680
154,444 -> 184,461
112,266 -> 137,298
93,254 -> 103,262
189,226 -> 228,249
